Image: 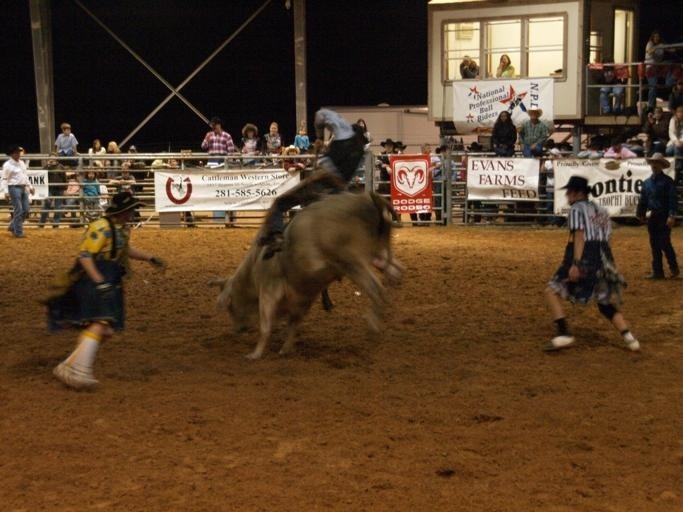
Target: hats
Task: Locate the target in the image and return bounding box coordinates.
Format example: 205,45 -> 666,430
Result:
6,144 -> 22,154
646,153 -> 670,169
381,138 -> 406,149
559,177 -> 588,191
283,145 -> 300,156
527,105 -> 542,116
105,192 -> 144,217
242,124 -> 258,137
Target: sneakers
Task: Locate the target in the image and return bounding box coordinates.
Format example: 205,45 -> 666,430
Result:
625,340 -> 639,350
542,336 -> 575,350
262,239 -> 282,258
646,273 -> 664,280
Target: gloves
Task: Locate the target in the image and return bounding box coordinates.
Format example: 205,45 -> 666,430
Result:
95,283 -> 112,300
148,256 -> 168,272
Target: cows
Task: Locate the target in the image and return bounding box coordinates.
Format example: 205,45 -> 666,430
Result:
208,191 -> 406,361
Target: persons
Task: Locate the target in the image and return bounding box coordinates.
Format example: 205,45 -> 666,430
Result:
377,138 -> 406,222
541,176 -> 641,354
39,124 -> 145,227
240,122 -> 281,168
636,153 -> 680,280
543,139 -> 572,224
410,143 -> 457,225
49,191 -> 168,389
2,146 -> 35,237
461,141 -> 484,222
151,149 -> 203,226
518,106 -> 549,158
577,142 -> 602,159
460,56 -> 479,79
281,104 -> 372,201
202,119 -> 234,166
497,55 -> 515,79
600,33 -> 683,183
492,111 -> 517,157
